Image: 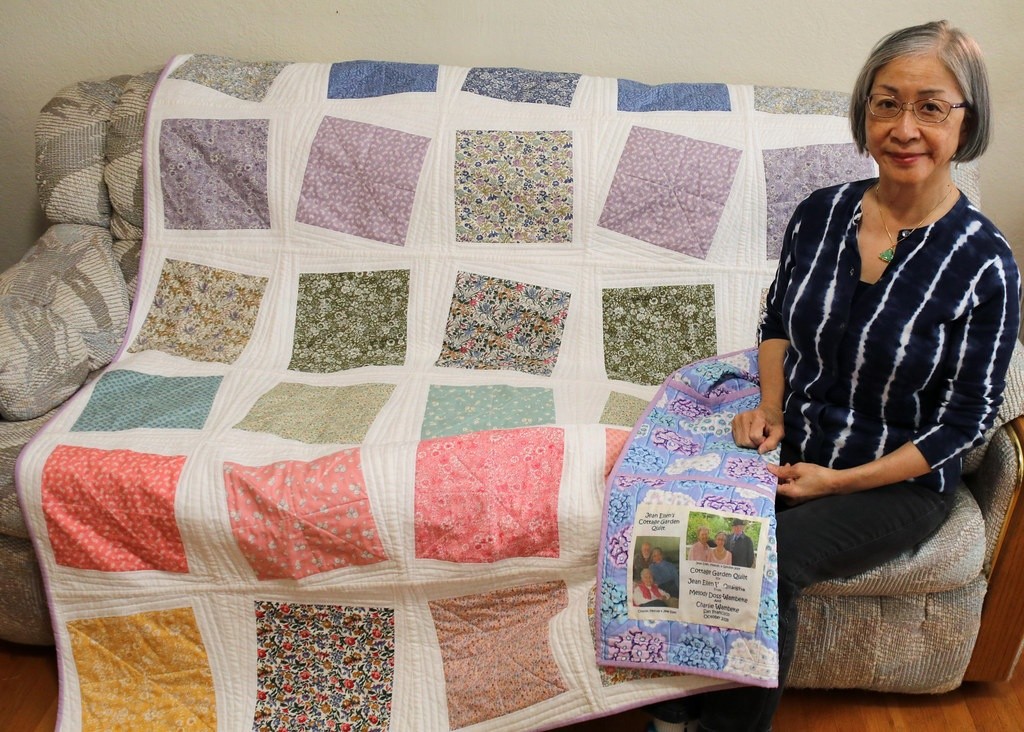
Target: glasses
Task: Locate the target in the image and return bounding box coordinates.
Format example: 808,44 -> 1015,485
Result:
865,93 -> 968,124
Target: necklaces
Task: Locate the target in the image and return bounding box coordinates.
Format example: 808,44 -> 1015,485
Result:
873,178 -> 952,263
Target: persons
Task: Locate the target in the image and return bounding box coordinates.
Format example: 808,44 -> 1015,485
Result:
657,21 -> 1024,732
633,542 -> 678,606
688,518 -> 754,568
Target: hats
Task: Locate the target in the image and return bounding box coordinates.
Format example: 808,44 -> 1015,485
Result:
731,517 -> 746,525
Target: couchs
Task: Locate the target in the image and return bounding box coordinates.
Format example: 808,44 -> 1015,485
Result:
0,53 -> 1024,701
